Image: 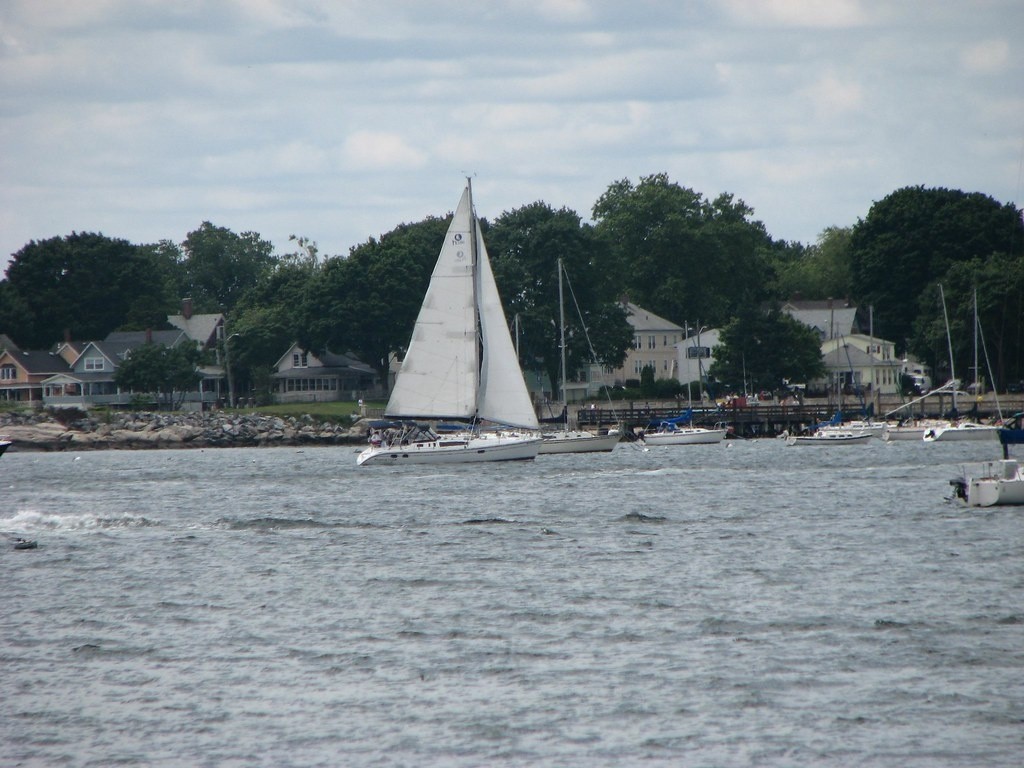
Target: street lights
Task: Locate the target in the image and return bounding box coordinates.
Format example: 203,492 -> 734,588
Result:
225,331 -> 242,410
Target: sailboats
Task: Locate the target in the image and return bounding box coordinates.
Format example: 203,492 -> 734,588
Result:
356,170 -> 545,469
818,282 -> 1024,509
447,254 -> 627,457
777,316 -> 876,448
638,322 -> 734,447
